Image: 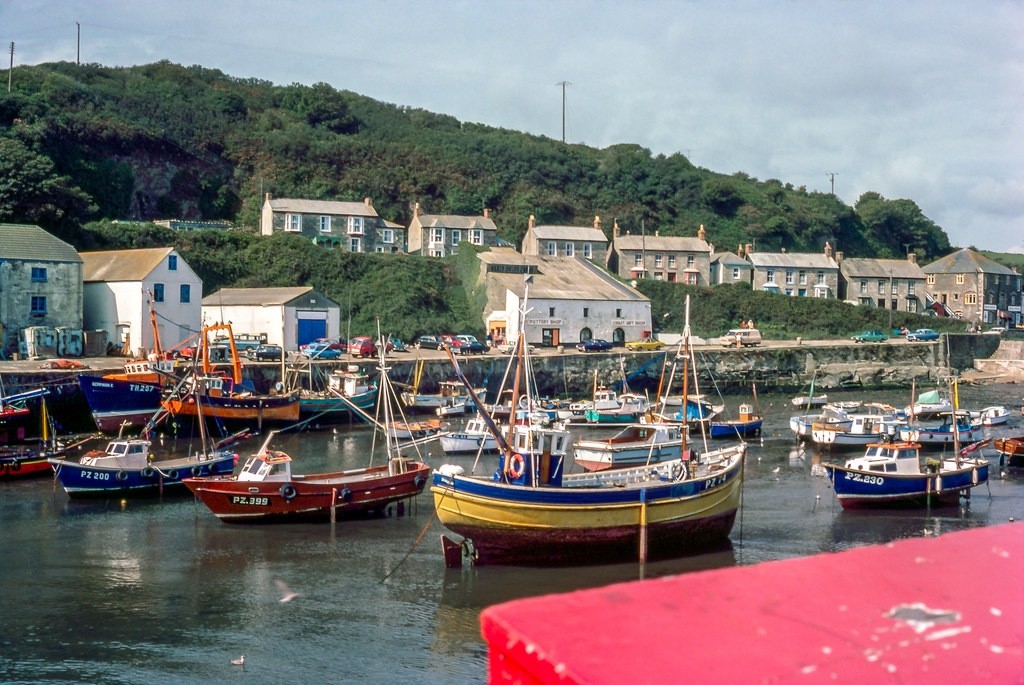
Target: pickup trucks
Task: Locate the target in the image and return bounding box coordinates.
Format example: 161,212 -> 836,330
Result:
496,341 -> 535,354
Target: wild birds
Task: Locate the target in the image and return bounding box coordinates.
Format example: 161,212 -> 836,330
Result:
923,528 -> 933,535
228,655 -> 247,665
757,436 -> 765,462
273,578 -> 302,604
772,467 -> 781,478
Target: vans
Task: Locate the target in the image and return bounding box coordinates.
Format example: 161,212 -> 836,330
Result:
719,329 -> 762,348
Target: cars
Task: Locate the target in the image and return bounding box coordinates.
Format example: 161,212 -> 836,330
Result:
414,334 -> 478,350
300,344 -> 342,360
906,329 -> 940,341
347,336 -> 378,357
982,327 -> 1006,335
850,330 -> 888,343
460,340 -> 491,354
624,336 -> 665,351
299,337 -> 344,351
576,339 -> 612,352
388,338 -> 406,351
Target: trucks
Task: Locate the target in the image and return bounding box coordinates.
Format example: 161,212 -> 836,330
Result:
212,333 -> 268,352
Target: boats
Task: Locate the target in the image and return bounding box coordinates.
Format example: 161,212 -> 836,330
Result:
74,287 -> 377,437
994,437 -> 1024,466
382,419 -> 451,439
789,379 -> 1011,453
401,352 -> 487,414
820,331 -> 994,507
792,369 -> 828,406
488,352 -> 764,440
46,338 -> 259,500
435,401 -> 466,416
181,317 -> 431,523
0,387 -> 66,478
430,265 -> 749,557
439,410 -> 516,455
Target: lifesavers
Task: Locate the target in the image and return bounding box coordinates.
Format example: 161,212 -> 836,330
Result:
415,475 -> 426,489
11,461 -> 22,471
0,461 -> 4,471
208,463 -> 220,474
279,483 -> 297,499
341,488 -> 353,503
140,466 -> 154,479
191,466 -> 202,478
672,464 -> 687,482
508,454 -> 526,478
115,470 -> 129,482
167,469 -> 179,481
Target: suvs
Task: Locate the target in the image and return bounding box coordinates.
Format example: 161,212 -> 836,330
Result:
256,344 -> 288,361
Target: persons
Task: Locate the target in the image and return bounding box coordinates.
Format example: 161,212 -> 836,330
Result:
735,333 -> 741,348
486,333 -> 493,347
